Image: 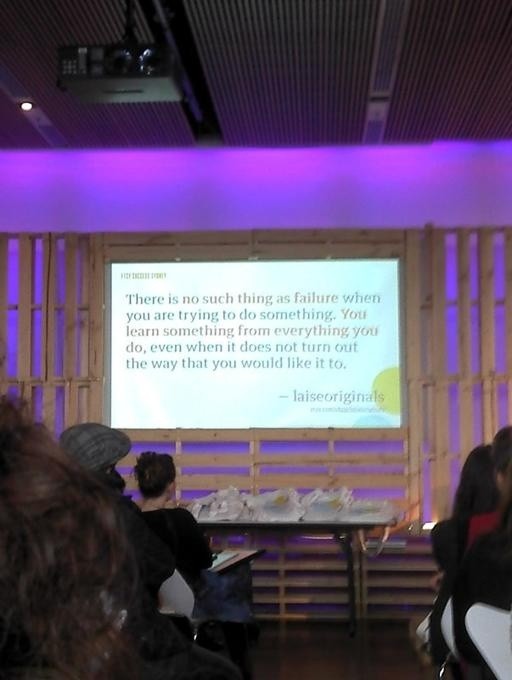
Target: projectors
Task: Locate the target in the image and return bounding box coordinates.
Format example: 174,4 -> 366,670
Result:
60,44 -> 184,103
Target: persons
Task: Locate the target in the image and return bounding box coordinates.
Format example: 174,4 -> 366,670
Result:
132,451 -> 212,592
54,424 -> 195,618
0,392 -> 243,680
417,423 -> 512,679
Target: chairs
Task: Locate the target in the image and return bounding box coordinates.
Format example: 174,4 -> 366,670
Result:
415,595 -> 512,680
156,569 -> 214,644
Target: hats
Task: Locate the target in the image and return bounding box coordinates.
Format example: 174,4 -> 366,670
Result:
58,423 -> 131,472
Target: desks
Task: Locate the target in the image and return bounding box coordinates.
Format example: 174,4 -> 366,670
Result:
196,510 -> 397,638
